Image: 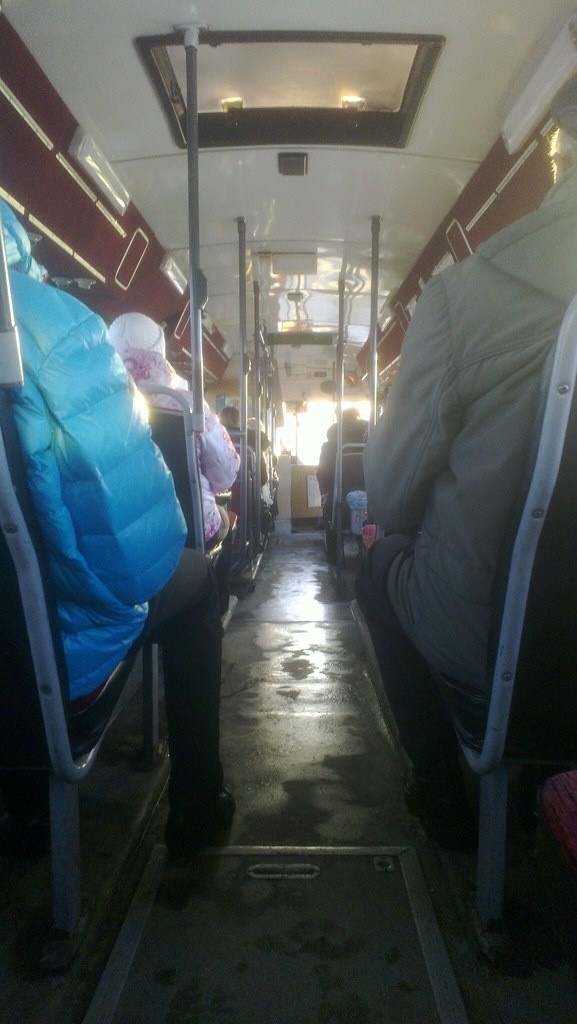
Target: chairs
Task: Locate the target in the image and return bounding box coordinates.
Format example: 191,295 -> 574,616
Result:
323,442 -> 365,534
377,305 -> 397,334
430,296 -> 577,956
0,261 -> 161,935
499,21 -> 577,155
228,428 -> 280,552
148,380 -> 225,563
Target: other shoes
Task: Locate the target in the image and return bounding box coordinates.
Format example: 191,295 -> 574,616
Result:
402,764 -> 479,847
166,786 -> 235,857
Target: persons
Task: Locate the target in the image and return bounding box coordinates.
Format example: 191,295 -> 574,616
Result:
218,406 -> 277,535
0,197 -> 239,844
356,76 -> 577,853
315,405 -> 371,524
288,402 -> 305,412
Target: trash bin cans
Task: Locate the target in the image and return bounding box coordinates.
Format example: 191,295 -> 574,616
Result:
349,491 -> 368,535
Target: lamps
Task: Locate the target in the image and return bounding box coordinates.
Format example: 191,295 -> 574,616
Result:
67,126 -> 131,215
158,253 -> 188,294
221,341 -> 233,360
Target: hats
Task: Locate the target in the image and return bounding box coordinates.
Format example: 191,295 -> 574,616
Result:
553,76 -> 577,137
107,313 -> 166,359
